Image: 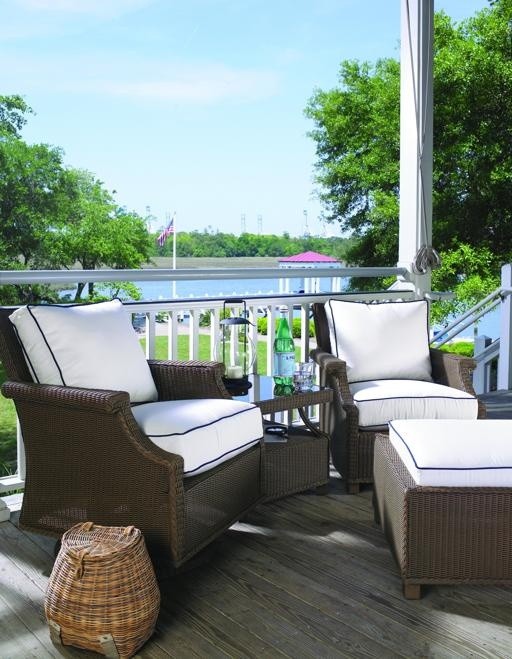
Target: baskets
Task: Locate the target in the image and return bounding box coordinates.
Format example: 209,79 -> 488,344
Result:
42,519 -> 163,659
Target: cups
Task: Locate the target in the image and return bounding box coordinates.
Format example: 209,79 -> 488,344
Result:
299,363 -> 314,391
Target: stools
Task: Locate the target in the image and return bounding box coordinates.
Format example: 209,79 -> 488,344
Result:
368,415 -> 512,601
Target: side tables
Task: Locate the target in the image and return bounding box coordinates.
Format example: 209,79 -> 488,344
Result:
225,372 -> 334,504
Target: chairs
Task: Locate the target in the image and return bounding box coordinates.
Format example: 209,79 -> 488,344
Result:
308,300 -> 488,496
1,294 -> 268,586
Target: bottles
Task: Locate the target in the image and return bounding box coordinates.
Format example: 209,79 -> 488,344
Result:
273,309 -> 295,385
274,387 -> 293,400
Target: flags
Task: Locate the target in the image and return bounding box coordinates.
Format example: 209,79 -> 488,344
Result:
156,217 -> 174,247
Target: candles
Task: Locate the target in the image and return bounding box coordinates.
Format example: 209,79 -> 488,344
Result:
229,364 -> 244,378
